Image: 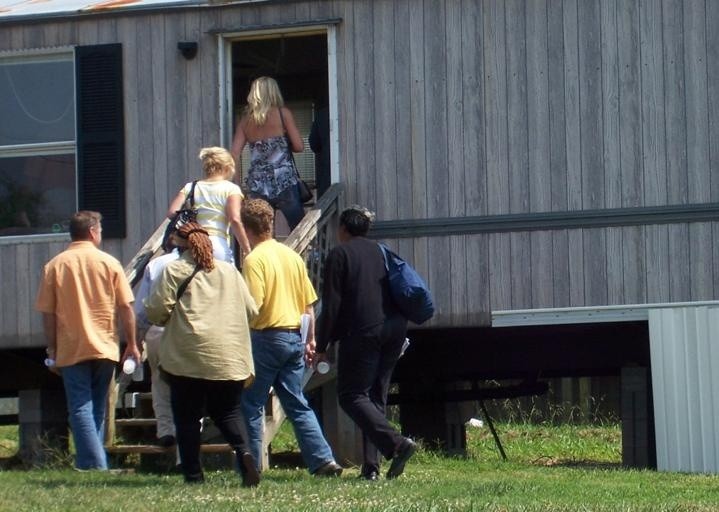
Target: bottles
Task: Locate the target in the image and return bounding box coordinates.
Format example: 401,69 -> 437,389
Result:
122,355 -> 136,374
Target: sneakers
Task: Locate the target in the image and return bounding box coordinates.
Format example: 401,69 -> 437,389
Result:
182,472 -> 203,483
316,462 -> 343,477
160,435 -> 174,446
239,452 -> 257,478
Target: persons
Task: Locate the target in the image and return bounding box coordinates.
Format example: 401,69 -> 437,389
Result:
230,76 -> 306,232
142,220 -> 260,488
235,199 -> 342,486
166,146 -> 252,268
134,224 -> 210,446
317,210 -> 417,479
35,211 -> 140,473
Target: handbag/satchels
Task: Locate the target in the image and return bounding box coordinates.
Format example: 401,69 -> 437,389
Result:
377,241 -> 435,325
161,180 -> 197,253
278,107 -> 313,205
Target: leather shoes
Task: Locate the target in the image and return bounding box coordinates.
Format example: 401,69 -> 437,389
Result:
387,438 -> 417,482
356,472 -> 376,481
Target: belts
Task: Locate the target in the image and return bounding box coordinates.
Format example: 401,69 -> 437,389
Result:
270,327 -> 299,333
